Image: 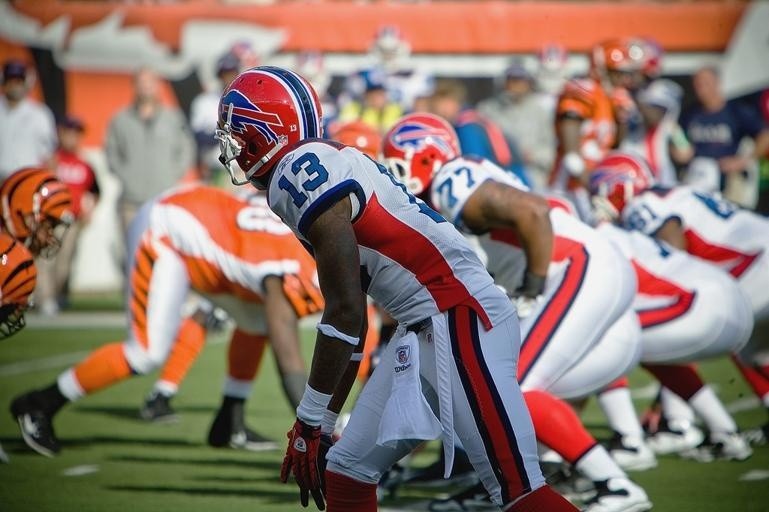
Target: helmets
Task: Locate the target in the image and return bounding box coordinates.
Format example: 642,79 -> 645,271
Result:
589,38 -> 654,88
214,67 -> 322,186
589,152 -> 657,220
1,168 -> 74,260
331,113 -> 461,196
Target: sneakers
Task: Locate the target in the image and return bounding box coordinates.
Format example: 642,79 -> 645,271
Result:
215,418 -> 279,449
542,421 -> 768,512
11,392 -> 61,458
140,394 -> 179,423
407,455 -> 494,511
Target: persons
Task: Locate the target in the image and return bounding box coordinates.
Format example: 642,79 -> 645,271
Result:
214,65 -> 580,512
1,47 -> 399,464
377,34 -> 768,511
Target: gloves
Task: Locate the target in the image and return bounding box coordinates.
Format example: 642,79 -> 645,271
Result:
278,416 -> 334,510
